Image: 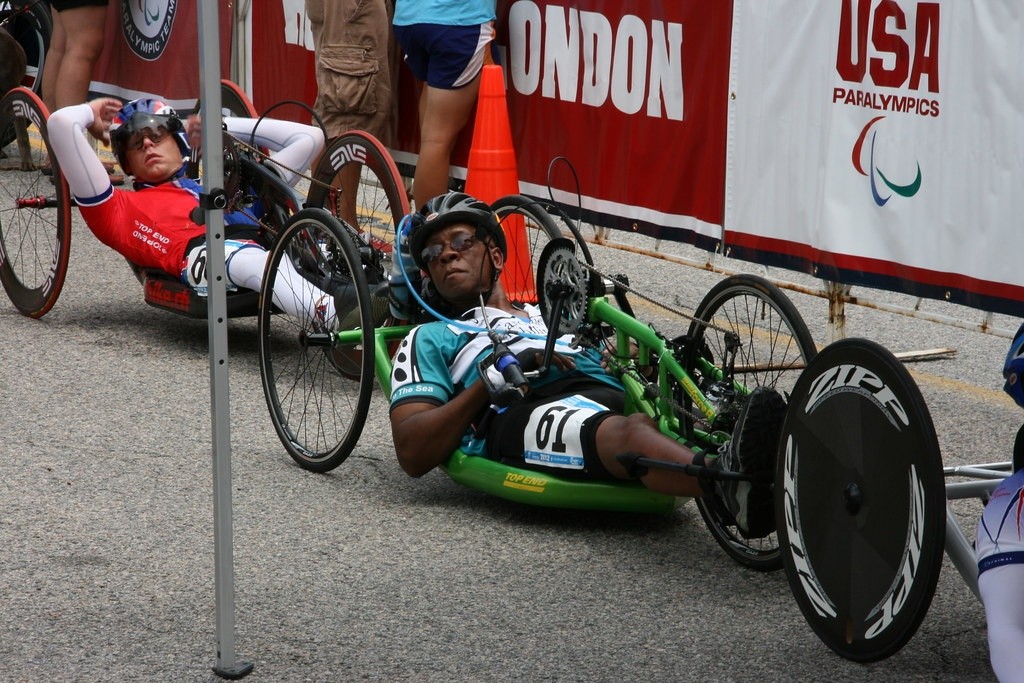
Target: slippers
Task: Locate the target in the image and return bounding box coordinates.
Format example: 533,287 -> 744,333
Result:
40,158 -> 125,187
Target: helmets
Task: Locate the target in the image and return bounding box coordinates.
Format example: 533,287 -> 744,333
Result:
110,98 -> 192,171
1002,322 -> 1024,406
409,192 -> 507,281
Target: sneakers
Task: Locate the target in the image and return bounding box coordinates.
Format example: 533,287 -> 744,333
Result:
707,385 -> 787,540
335,280 -> 390,333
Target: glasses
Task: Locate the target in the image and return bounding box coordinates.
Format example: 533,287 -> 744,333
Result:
416,228 -> 484,264
119,114 -> 182,151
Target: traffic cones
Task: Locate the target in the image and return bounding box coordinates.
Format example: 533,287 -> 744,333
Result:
463,64 -> 539,305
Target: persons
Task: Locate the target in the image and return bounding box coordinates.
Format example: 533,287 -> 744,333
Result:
975,321 -> 1024,683
47,97 -> 388,350
304,0 -> 499,261
389,191 -> 788,537
41,0 -> 125,186
